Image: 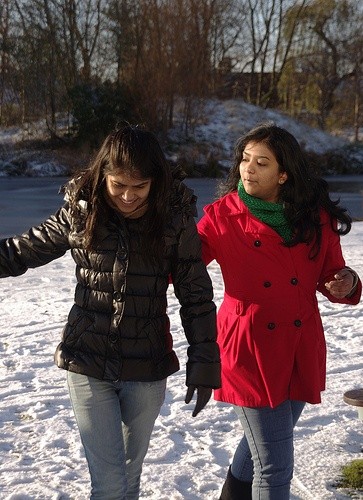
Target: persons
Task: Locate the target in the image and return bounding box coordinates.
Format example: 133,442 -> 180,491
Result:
168,123 -> 363,500
1,122 -> 221,499
